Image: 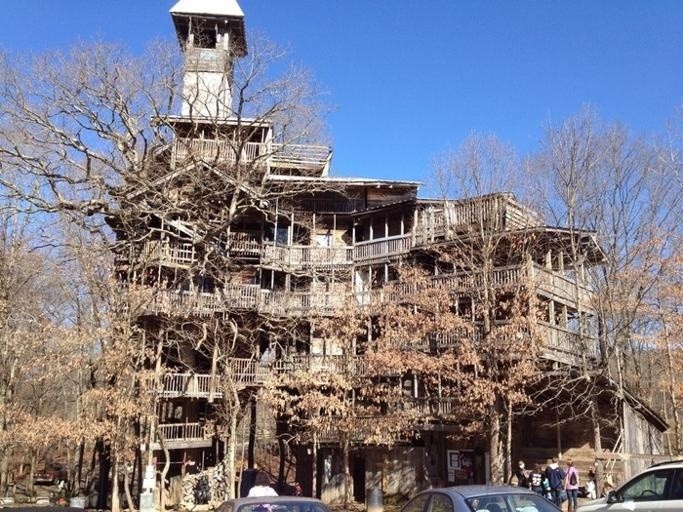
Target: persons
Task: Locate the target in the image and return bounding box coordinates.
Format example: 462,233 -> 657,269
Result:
247,472 -> 278,512
516,458 -> 580,512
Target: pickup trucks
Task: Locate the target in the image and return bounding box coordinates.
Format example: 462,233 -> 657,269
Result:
34,464 -> 67,484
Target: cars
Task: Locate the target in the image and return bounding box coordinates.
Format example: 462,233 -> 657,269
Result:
215,496 -> 328,512
399,484 -> 562,512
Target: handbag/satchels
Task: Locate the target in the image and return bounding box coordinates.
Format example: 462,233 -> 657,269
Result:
570,472 -> 577,485
532,474 -> 542,486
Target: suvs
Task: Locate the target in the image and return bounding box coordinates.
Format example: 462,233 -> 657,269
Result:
576,460 -> 683,512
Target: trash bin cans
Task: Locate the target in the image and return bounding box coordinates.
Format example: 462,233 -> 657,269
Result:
367,489 -> 384,512
